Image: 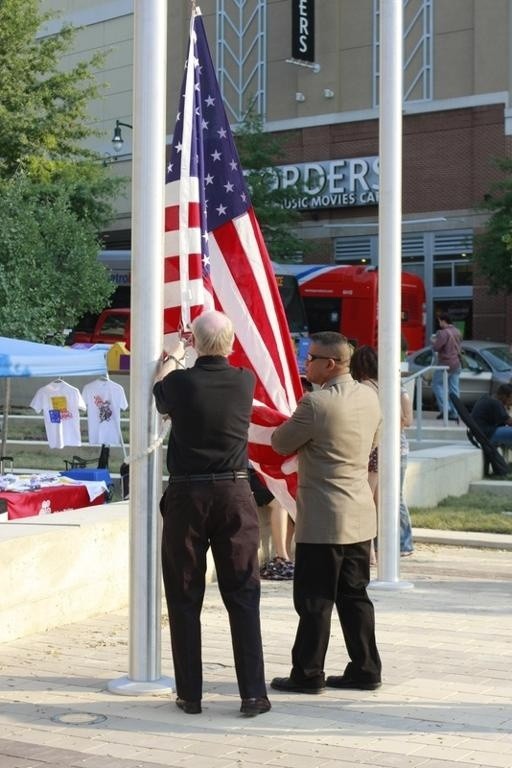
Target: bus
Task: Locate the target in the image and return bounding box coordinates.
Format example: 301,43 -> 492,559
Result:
273,264 -> 425,359
70,248 -> 310,405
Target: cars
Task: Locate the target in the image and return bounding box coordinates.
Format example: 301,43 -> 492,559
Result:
403,336 -> 511,415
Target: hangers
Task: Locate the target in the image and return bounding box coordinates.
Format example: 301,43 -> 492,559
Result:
102,373 -> 114,383
52,375 -> 65,384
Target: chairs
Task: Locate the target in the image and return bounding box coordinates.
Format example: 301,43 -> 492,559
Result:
62,445 -> 112,469
0,454 -> 15,476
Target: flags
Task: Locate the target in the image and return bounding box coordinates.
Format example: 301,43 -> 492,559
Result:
164,7 -> 307,522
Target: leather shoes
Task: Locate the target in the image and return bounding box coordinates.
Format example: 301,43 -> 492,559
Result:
240,696 -> 271,715
175,697 -> 202,713
270,677 -> 324,694
326,676 -> 381,690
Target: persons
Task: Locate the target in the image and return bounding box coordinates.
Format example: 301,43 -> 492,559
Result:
155,309 -> 270,715
468,382 -> 512,477
348,343 -> 384,568
429,313 -> 462,420
269,330 -> 383,693
391,383 -> 412,556
253,478 -> 294,577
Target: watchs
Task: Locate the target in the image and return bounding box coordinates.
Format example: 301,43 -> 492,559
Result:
163,354 -> 178,368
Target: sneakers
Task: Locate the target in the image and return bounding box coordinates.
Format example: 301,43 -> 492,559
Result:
260,557 -> 294,580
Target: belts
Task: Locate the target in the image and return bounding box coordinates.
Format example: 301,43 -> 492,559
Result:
172,471 -> 249,483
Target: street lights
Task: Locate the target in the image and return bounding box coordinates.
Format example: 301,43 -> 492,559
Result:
112,117 -> 133,150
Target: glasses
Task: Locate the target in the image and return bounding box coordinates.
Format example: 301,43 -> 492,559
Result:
307,353 -> 341,362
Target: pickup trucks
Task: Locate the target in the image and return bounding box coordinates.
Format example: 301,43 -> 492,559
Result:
72,309 -> 130,349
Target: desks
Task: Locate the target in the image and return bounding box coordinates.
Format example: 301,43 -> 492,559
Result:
62,466 -> 112,482
0,474 -> 106,521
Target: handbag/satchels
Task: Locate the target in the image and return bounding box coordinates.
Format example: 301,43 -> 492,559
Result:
457,351 -> 470,368
